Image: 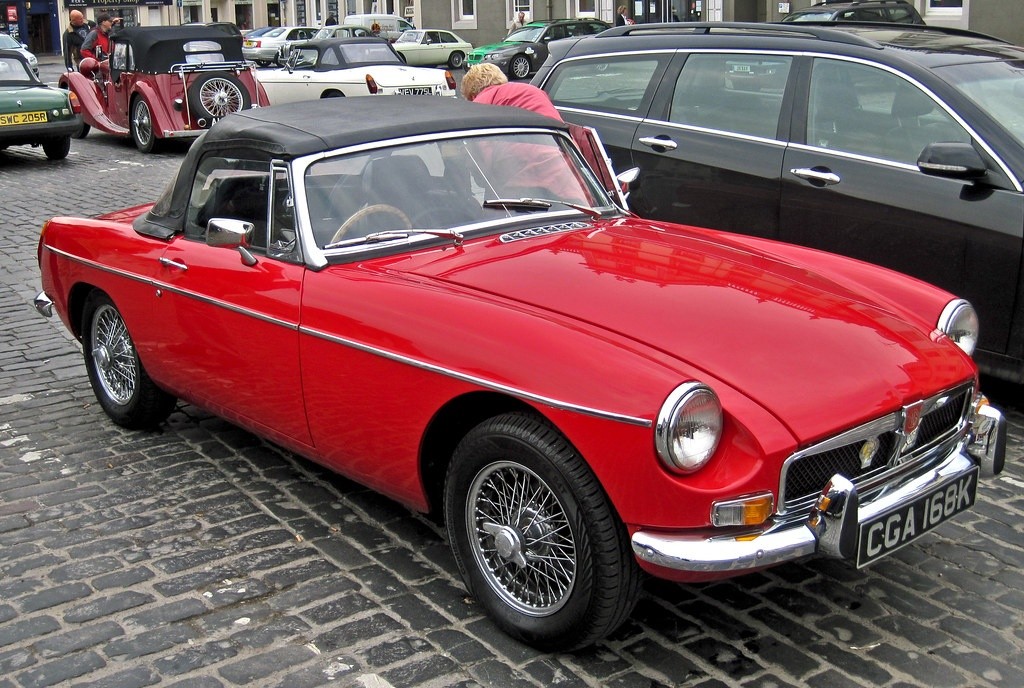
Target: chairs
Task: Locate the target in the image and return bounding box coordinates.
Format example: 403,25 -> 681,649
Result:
362,154 -> 441,231
817,90 -> 885,155
890,86 -> 957,165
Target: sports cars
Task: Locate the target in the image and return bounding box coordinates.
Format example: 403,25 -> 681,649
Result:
0,50 -> 83,161
35,93 -> 1010,659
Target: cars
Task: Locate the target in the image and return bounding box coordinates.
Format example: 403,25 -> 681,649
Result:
247,35 -> 457,107
0,32 -> 42,79
56,26 -> 273,153
179,20 -> 244,50
238,24 -> 475,69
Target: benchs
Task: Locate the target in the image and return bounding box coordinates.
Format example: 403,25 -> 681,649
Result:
193,175 -> 364,248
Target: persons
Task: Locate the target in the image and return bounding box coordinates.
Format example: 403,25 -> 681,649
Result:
325,12 -> 336,38
460,64 -> 601,220
507,12 -> 527,37
63,10 -> 124,73
80,14 -> 115,61
617,5 -> 635,25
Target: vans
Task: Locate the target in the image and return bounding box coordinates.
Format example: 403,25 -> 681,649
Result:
343,13 -> 417,41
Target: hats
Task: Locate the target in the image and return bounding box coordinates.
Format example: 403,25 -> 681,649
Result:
97,13 -> 113,23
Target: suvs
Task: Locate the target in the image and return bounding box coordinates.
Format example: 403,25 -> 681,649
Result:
462,17 -> 612,81
529,16 -> 1023,407
719,0 -> 927,110
